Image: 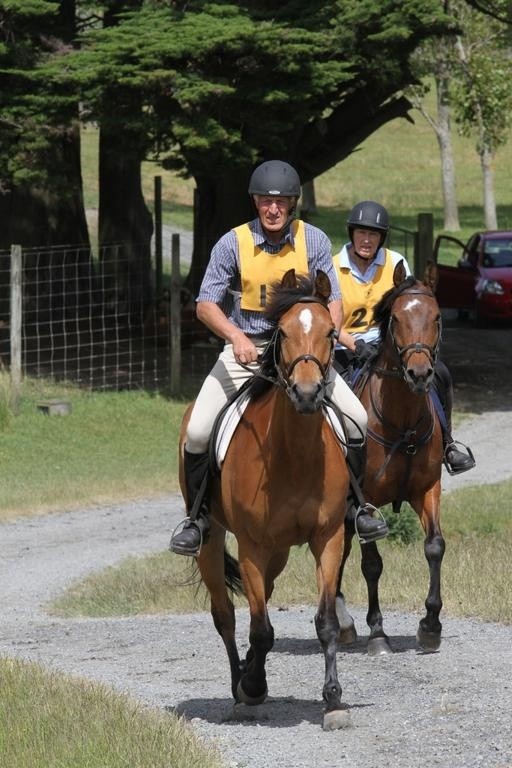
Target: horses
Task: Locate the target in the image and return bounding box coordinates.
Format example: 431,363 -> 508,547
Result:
334,258 -> 448,661
178,268 -> 352,732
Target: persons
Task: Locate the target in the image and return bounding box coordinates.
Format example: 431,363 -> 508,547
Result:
330,200 -> 474,471
168,158 -> 388,554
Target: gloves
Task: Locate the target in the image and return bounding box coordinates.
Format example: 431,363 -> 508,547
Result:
354,339 -> 375,359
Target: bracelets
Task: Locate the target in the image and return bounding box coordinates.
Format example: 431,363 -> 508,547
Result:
334,329 -> 339,341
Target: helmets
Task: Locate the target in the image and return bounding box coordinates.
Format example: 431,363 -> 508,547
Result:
247,160 -> 301,198
347,201 -> 390,231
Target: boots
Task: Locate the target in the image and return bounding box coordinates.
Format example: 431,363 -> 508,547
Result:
439,391 -> 473,467
170,443 -> 211,551
346,438 -> 388,539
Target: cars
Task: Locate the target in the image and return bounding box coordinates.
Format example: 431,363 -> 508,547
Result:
433,230 -> 512,327
